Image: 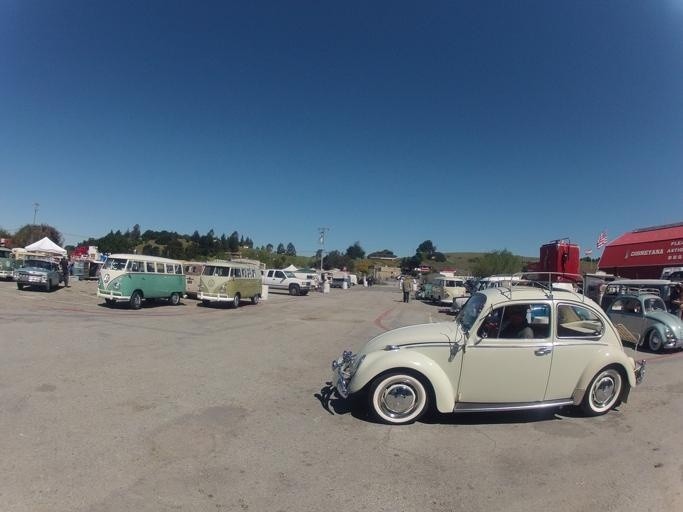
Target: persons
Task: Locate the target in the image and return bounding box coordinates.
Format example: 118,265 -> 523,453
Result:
402,276 -> 412,303
59,255 -> 70,287
670,284 -> 683,320
502,304 -> 533,338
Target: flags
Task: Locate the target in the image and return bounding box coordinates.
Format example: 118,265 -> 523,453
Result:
597,230 -> 607,249
583,248 -> 592,256
